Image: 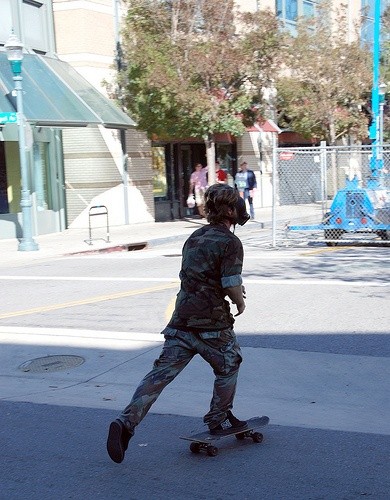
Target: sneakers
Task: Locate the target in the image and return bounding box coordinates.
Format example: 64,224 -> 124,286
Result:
208,410 -> 250,436
107,419 -> 134,463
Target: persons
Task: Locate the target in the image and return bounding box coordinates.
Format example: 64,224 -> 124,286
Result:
236,160 -> 259,222
105,185 -> 250,464
188,162 -> 210,215
215,161 -> 229,185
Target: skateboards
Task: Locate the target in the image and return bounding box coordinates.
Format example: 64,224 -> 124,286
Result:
178,415 -> 269,457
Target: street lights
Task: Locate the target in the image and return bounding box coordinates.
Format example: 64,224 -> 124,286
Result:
3,28 -> 40,251
